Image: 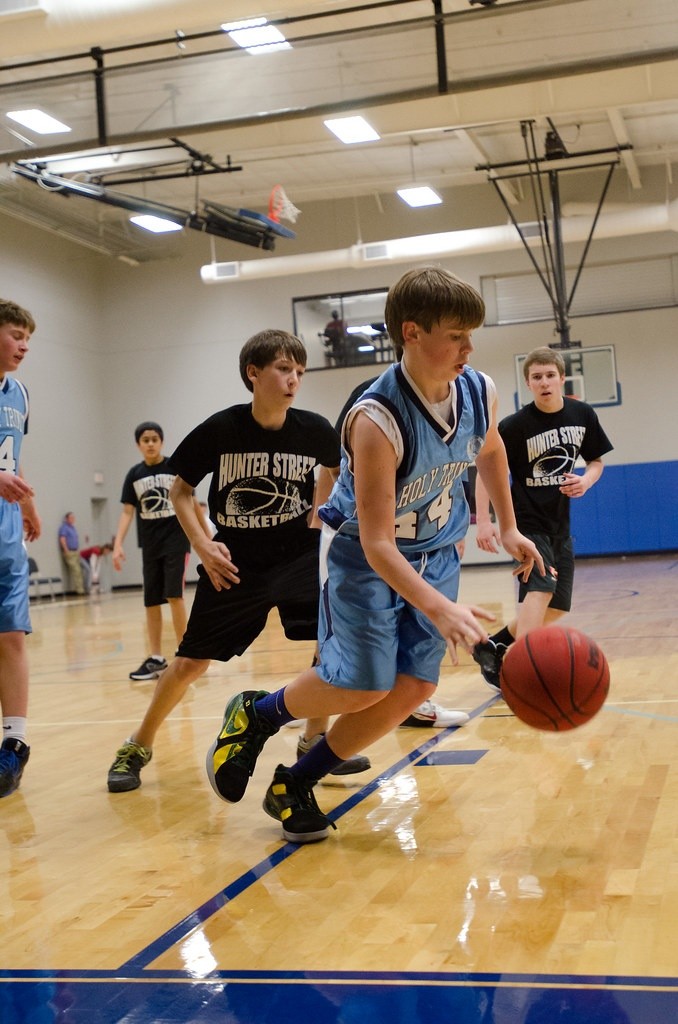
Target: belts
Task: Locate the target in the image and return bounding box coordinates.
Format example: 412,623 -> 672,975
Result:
69,549 -> 77,551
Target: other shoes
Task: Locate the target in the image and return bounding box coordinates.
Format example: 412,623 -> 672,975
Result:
77,592 -> 89,596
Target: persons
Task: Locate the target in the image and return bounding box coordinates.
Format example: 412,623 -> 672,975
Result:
474,348 -> 615,693
308,344 -> 471,727
326,312 -> 349,363
0,300 -> 37,797
107,329 -> 371,792
111,421 -> 212,681
205,265 -> 547,844
59,502 -> 209,597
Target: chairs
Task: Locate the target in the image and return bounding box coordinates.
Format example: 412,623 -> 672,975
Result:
26,556 -> 62,604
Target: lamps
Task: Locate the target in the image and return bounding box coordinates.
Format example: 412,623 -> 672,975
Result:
396,137 -> 443,207
128,179 -> 182,234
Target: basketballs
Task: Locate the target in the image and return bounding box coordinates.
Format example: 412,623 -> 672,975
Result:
500,623 -> 612,735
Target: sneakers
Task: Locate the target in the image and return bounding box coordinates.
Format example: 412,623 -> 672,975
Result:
206,690 -> 280,804
400,701 -> 470,728
297,732 -> 371,775
0,738 -> 30,798
107,737 -> 152,791
130,656 -> 168,680
471,634 -> 508,688
263,764 -> 329,842
